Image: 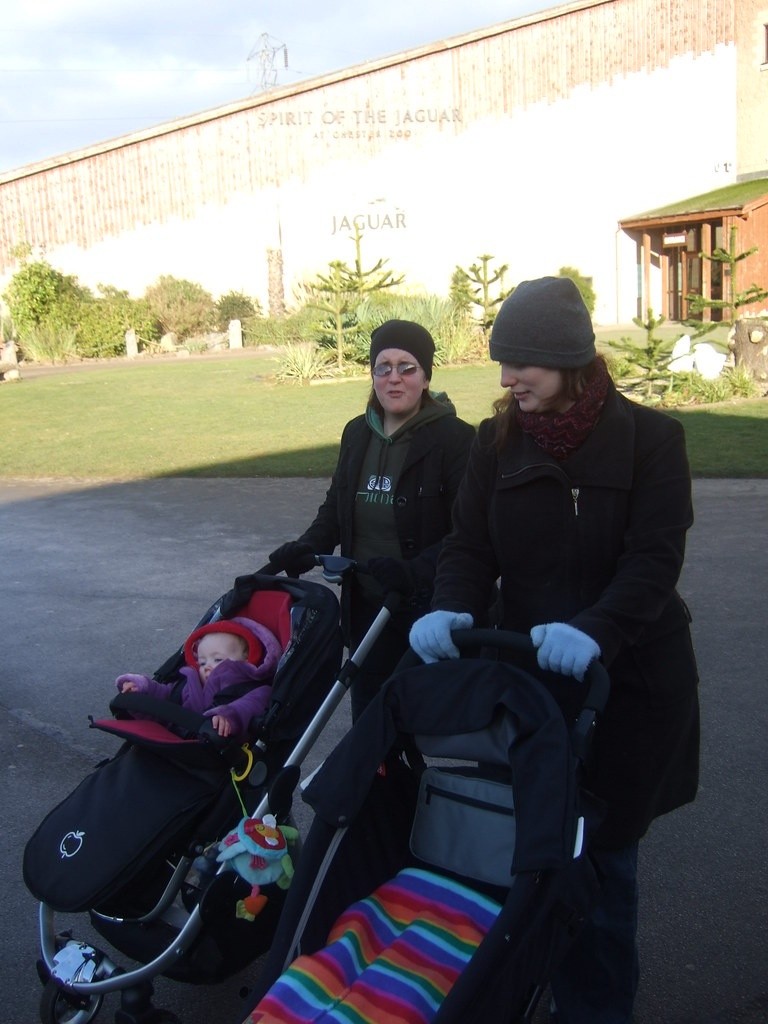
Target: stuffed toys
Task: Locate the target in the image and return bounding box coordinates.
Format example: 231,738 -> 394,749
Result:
216,815 -> 298,921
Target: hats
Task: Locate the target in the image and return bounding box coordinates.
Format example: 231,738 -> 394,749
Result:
490,276 -> 597,369
370,319 -> 436,378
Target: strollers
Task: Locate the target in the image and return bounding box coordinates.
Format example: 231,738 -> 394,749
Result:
228,626 -> 611,1021
18,550 -> 401,1024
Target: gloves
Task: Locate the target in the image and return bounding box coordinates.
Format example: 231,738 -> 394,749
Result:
269,540 -> 317,578
530,621 -> 600,684
366,554 -> 408,595
408,610 -> 474,664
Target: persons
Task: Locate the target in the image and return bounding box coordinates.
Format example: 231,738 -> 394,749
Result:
409,276 -> 699,1023
117,618 -> 277,737
269,319 -> 477,725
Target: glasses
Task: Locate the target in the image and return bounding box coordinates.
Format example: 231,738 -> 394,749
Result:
373,360 -> 424,376
